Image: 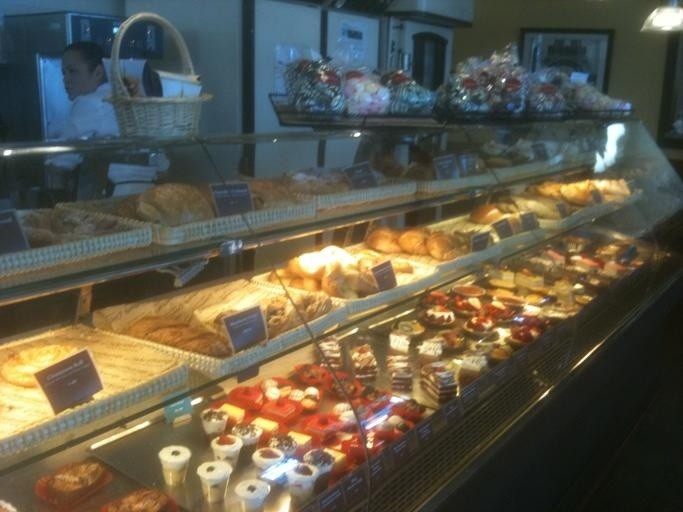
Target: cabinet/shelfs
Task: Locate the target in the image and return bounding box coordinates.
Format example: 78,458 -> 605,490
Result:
0,105 -> 682,512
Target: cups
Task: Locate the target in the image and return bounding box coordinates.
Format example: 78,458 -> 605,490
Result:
155,408 -> 336,512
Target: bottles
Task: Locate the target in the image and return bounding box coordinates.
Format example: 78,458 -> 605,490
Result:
414,32 -> 448,93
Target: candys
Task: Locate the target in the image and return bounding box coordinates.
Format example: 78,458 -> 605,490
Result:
345,78 -> 390,114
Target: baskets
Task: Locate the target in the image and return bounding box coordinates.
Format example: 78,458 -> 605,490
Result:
101,12 -> 213,140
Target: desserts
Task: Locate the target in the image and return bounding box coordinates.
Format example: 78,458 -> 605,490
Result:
158,408 -> 335,511
397,264 -> 589,359
318,330 -> 490,407
206,361 -> 425,486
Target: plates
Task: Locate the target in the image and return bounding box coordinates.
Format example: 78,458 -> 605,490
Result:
418,284 -> 591,348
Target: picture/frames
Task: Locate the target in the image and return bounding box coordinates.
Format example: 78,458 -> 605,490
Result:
514,24 -> 614,96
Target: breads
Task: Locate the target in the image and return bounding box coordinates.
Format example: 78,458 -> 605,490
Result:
19,152 -> 523,244
364,226 -> 457,260
94,276 -> 333,357
268,244 -> 415,298
49,462 -> 167,512
2,340 -> 77,385
425,178 -> 630,291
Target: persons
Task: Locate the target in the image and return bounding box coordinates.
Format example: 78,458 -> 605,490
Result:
46,41 -> 163,199
